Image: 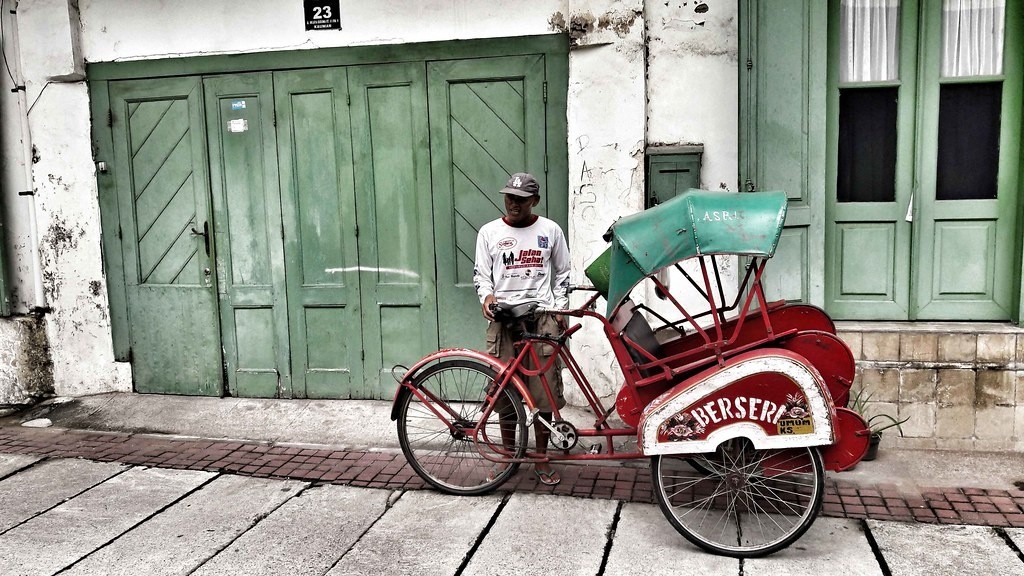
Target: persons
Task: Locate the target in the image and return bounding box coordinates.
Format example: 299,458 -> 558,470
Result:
472,173 -> 572,486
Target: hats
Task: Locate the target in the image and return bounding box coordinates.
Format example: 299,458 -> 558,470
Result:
499,173 -> 539,197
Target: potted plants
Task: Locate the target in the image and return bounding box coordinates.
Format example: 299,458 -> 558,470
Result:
847,387 -> 911,461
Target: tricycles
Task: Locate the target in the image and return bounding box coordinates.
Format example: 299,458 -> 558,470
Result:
387,186 -> 869,558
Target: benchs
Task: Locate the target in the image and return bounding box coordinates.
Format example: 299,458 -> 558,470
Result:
619,311 -> 681,397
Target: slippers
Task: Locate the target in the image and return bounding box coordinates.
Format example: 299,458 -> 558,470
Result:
486,466 -> 504,482
534,469 -> 560,484
